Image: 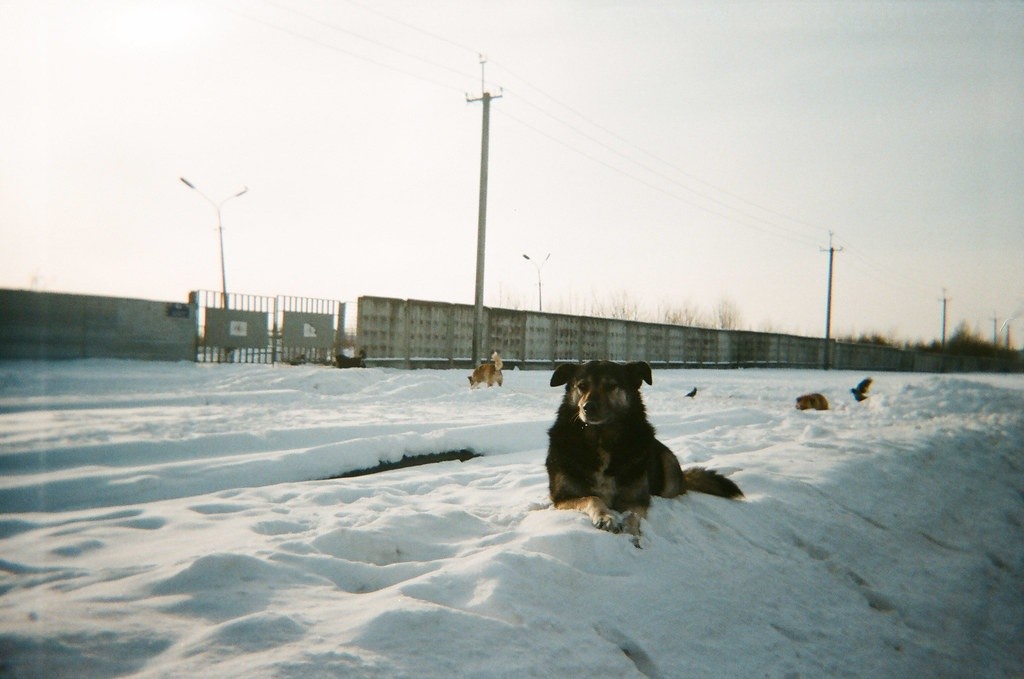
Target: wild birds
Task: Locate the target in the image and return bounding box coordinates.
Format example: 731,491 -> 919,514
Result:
685,387 -> 697,399
851,377 -> 873,402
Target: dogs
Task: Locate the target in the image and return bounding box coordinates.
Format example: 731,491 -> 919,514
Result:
795,393 -> 829,411
544,360 -> 745,549
336,349 -> 367,368
467,351 -> 504,390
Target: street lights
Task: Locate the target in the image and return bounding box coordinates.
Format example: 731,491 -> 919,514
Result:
180,175 -> 248,310
523,252 -> 551,311
